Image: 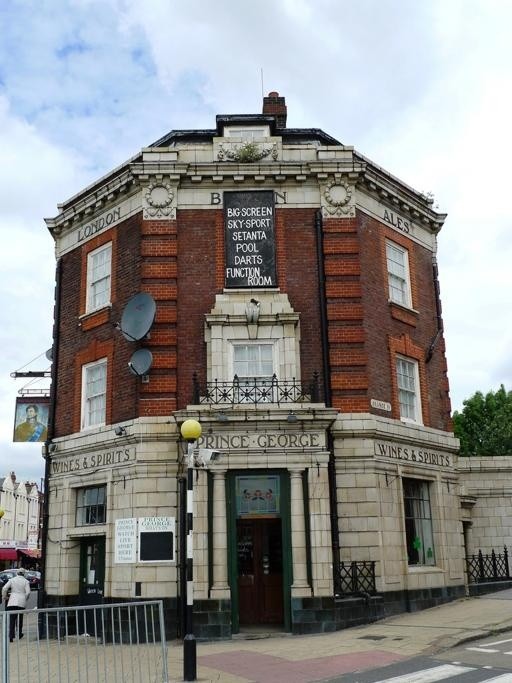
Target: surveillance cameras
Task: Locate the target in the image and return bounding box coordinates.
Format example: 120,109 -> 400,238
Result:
198,449 -> 222,464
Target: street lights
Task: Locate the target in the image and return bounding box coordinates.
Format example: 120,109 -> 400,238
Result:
180,418 -> 202,680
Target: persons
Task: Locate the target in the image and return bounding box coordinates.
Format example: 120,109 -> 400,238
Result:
14,405 -> 48,442
2,567 -> 31,642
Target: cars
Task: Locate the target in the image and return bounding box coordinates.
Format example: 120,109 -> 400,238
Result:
0,568 -> 41,603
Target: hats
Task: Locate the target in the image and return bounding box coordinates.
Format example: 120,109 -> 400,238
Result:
17,568 -> 25,574
